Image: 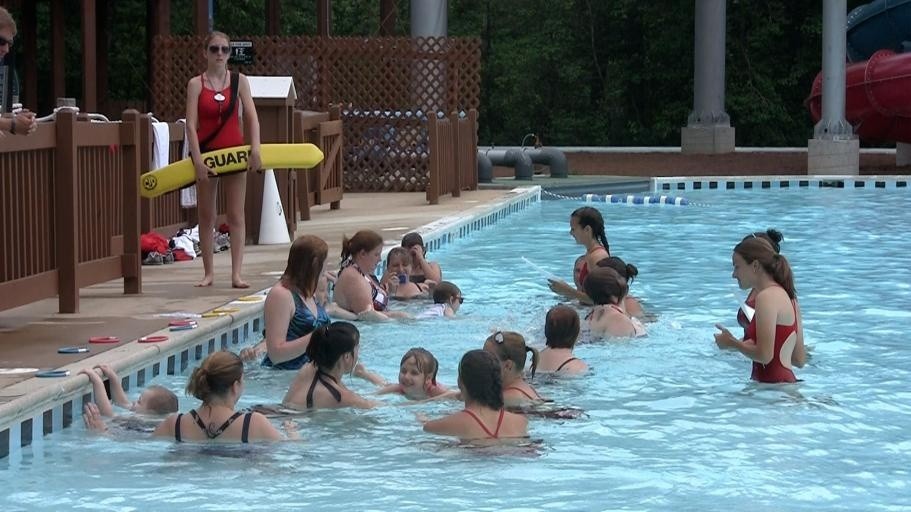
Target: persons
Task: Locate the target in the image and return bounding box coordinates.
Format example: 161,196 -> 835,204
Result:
316,228 -> 464,320
284,321 -> 377,410
82,349 -> 288,439
713,236 -> 799,382
546,207 -> 611,306
77,364 -> 179,433
483,331 -> 539,399
0,6 -> 38,136
537,304 -> 587,373
581,256 -> 640,337
384,347 -> 453,398
186,31 -> 261,289
738,227 -> 806,368
264,234 -> 332,369
423,350 -> 529,436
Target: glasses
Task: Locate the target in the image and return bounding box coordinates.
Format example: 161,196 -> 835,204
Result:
206,44 -> 232,55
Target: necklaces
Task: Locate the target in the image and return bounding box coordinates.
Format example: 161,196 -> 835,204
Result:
207,70 -> 229,102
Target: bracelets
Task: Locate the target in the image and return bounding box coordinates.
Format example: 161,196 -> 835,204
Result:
10,117 -> 17,135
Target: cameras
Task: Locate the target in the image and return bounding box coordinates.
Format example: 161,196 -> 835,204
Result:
399,276 -> 406,283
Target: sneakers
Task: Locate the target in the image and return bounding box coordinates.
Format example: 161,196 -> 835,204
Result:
192,232 -> 231,255
142,249 -> 175,265
